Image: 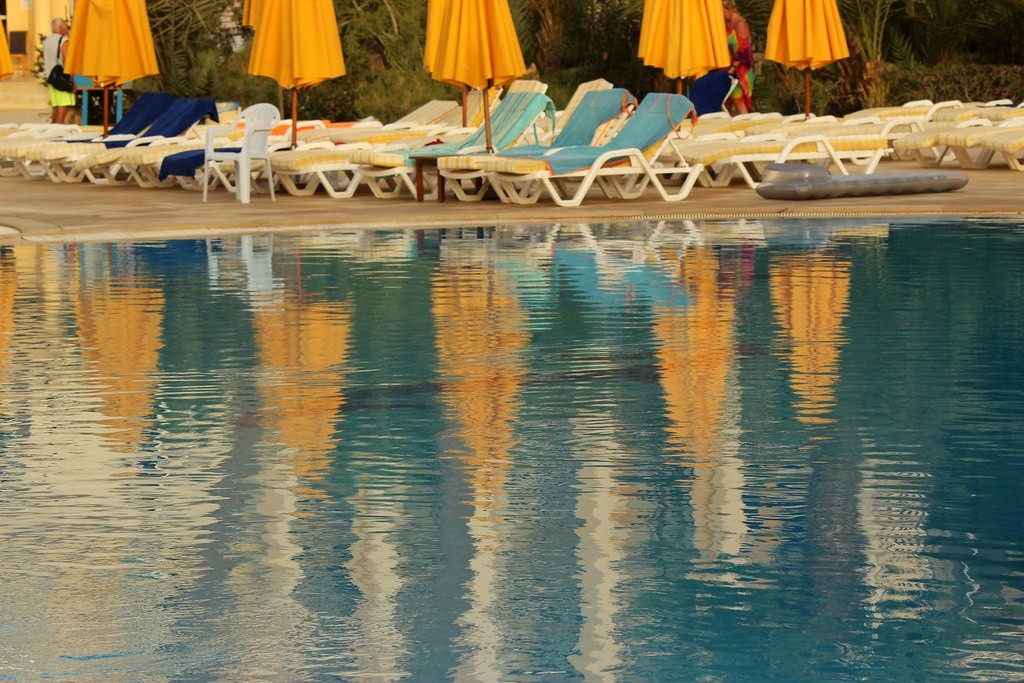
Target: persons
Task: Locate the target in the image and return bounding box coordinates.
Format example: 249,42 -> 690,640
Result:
495,62 -> 540,90
44,18 -> 77,123
721,0 -> 755,114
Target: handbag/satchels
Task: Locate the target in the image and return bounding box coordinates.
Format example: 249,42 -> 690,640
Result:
47,64 -> 73,93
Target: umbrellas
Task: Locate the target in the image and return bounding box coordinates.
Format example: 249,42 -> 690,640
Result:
241,0 -> 345,147
765,0 -> 850,117
423,0 -> 526,151
63,0 -> 160,135
638,1 -> 731,96
1,19 -> 14,82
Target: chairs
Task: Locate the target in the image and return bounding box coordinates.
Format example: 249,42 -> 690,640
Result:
8,29 -> 28,77
0,77 -> 1024,208
